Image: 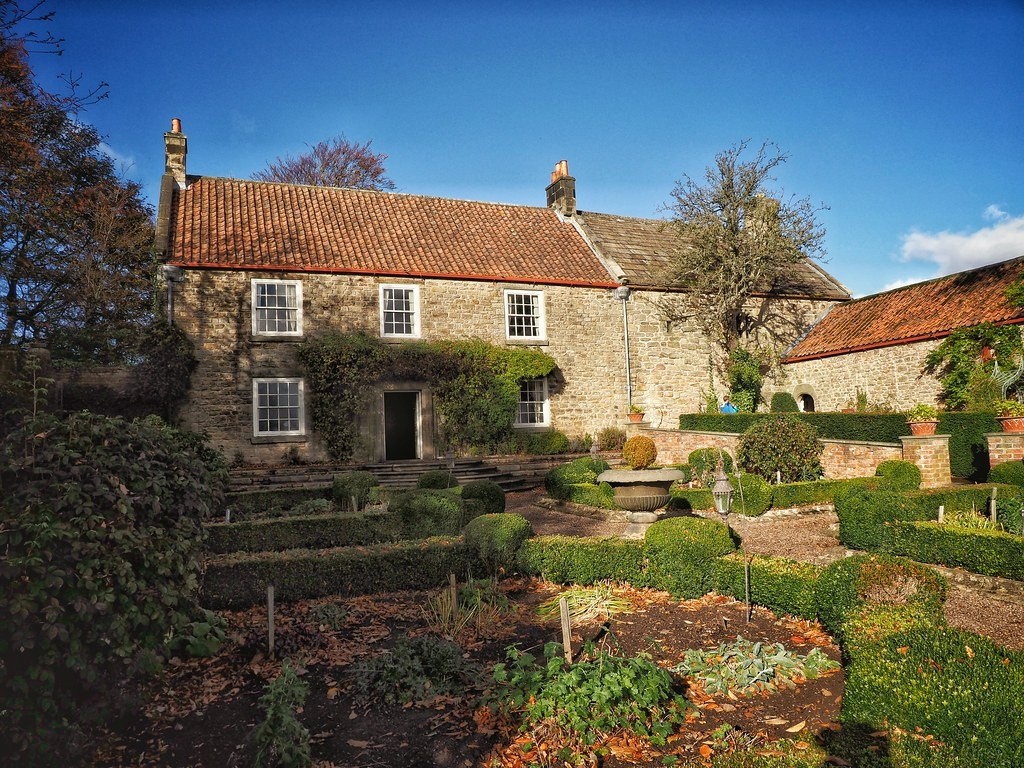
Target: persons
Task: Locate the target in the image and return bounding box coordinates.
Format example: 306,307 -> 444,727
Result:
718,396 -> 739,414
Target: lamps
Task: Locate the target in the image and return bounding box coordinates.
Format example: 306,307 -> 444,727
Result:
443,448 -> 456,470
711,466 -> 735,517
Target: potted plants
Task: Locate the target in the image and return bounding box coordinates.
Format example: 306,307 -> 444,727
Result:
903,401 -> 940,437
627,403 -> 646,422
993,400 -> 1024,432
